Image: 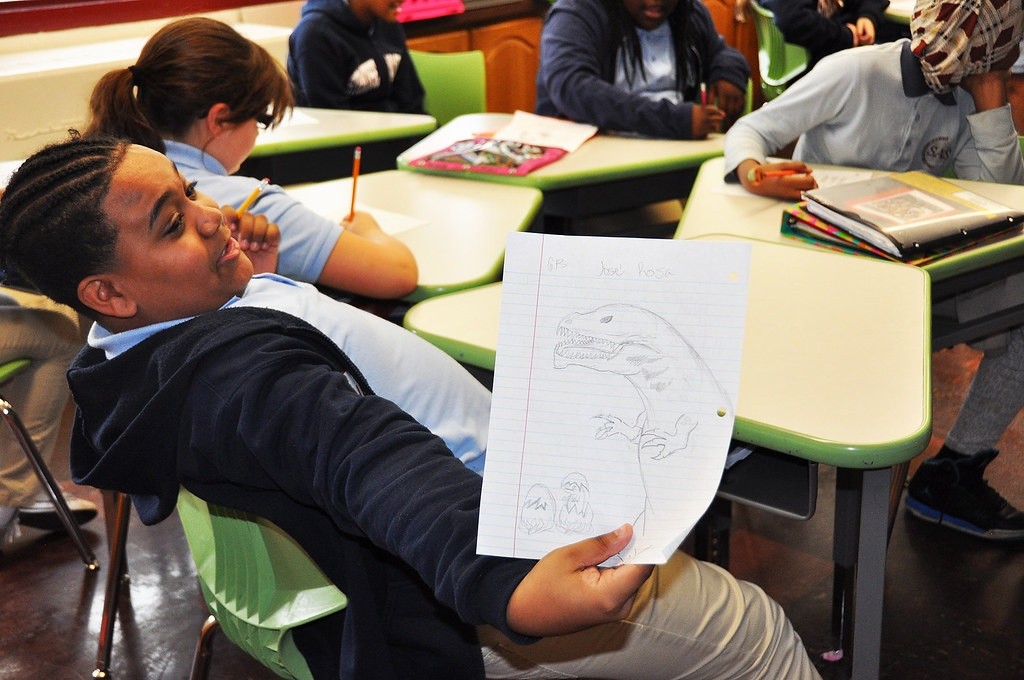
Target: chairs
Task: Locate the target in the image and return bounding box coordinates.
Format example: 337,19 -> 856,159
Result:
0,358 -> 220,680
409,49 -> 488,125
177,486 -> 350,680
750,0 -> 810,100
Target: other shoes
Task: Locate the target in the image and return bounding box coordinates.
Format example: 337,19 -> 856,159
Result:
21,481 -> 99,527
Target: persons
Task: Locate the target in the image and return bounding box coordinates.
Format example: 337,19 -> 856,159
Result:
288,0 -> 425,113
0,286 -> 100,539
538,0 -> 751,138
725,0 -> 1024,538
1,138 -> 823,679
77,18 -> 419,300
759,0 -> 891,62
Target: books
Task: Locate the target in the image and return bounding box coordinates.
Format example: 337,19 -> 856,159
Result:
780,169 -> 1024,266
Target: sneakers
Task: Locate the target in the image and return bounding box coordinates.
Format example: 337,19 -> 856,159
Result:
905,448 -> 1024,539
719,439 -> 818,520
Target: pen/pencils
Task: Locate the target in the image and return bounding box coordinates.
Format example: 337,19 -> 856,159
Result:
700,84 -> 707,104
747,165 -> 799,186
241,177 -> 269,213
350,147 -> 363,218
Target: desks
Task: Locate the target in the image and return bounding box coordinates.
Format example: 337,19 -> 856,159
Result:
249,106 -> 1024,680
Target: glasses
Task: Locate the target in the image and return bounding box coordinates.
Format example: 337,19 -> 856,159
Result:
253,112 -> 273,129
